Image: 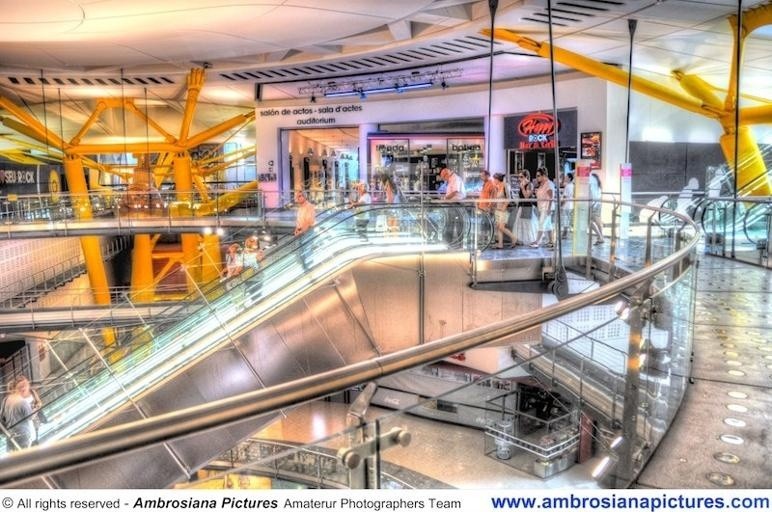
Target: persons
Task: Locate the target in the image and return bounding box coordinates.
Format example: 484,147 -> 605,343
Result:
638,168 -> 725,224
0,375 -> 43,451
216,242 -> 244,309
435,162 -> 606,250
3,381 -> 41,452
293,189 -> 317,274
242,235 -> 266,304
348,180 -> 371,241
382,175 -> 403,235
